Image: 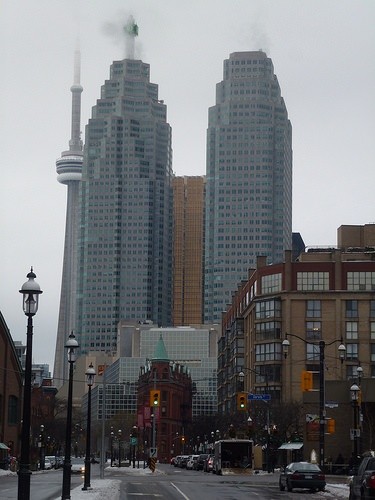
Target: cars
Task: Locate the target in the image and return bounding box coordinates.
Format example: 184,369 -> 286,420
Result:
37,455 -> 100,474
350,451 -> 375,500
170,453 -> 214,476
279,462 -> 325,492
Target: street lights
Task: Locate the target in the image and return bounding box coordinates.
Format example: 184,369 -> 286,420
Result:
182,430 -> 220,455
247,416 -> 252,441
356,366 -> 363,388
82,361 -> 94,490
111,425 -> 138,468
230,424 -> 233,439
349,382 -> 360,461
39,424 -> 44,469
64,330 -> 80,500
238,367 -> 272,473
17,266 -> 43,500
281,331 -> 347,467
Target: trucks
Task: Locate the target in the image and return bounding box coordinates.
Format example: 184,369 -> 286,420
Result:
213,439 -> 255,476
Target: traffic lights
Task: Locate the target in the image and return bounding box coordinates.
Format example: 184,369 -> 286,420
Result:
271,424 -> 279,432
149,389 -> 161,407
237,393 -> 247,412
